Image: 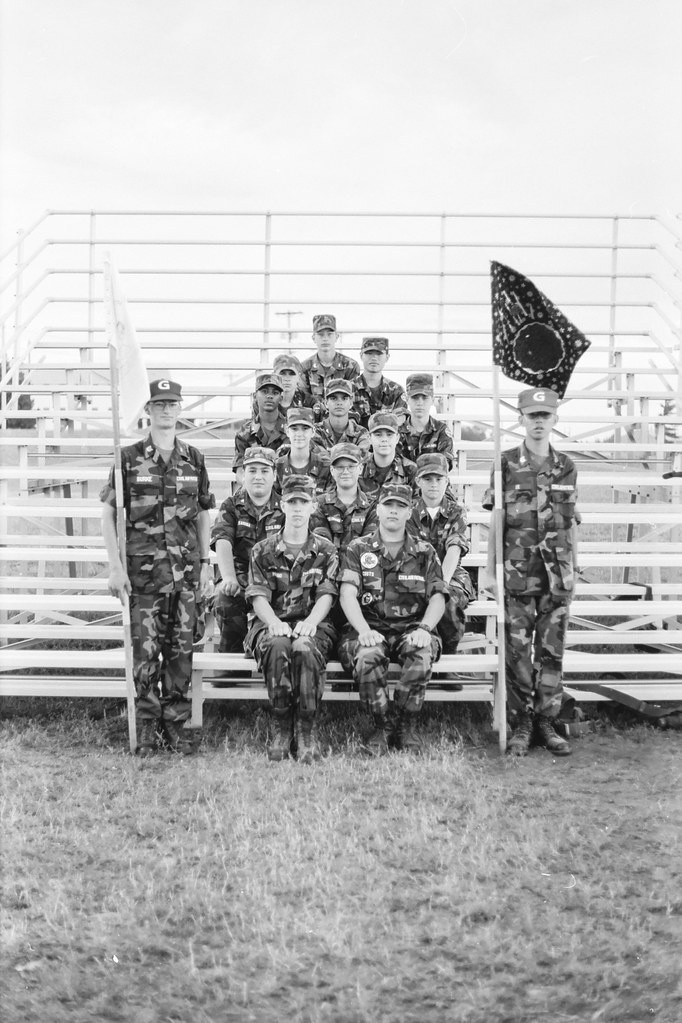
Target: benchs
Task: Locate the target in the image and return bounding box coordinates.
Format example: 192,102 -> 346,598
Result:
1,310 -> 682,751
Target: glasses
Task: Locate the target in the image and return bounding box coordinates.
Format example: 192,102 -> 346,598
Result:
333,464 -> 360,473
150,402 -> 181,410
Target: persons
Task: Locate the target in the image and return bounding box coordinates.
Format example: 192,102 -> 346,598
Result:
99,380 -> 216,757
274,408 -> 337,495
336,483 -> 450,756
272,353 -> 316,416
308,443 -> 379,548
395,373 -> 453,472
408,452 -> 477,691
315,378 -> 372,455
349,337 -> 407,414
232,374 -> 291,486
358,412 -> 416,495
244,474 -> 339,761
482,387 -> 581,757
211,447 -> 285,687
302,314 -> 360,396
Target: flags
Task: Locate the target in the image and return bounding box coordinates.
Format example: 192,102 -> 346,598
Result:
490,260 -> 591,400
99,265 -> 152,433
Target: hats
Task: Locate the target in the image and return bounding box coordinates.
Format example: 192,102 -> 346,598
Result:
313,314 -> 337,332
281,474 -> 316,502
242,447 -> 276,466
361,337 -> 389,354
378,482 -> 413,506
285,408 -> 316,429
148,380 -> 183,402
518,389 -> 558,414
324,380 -> 353,396
406,373 -> 433,396
256,374 -> 285,390
330,443 -> 360,462
416,453 -> 448,478
367,412 -> 398,433
274,354 -> 301,377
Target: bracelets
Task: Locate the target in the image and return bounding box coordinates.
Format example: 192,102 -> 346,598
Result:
418,624 -> 430,632
200,558 -> 210,565
572,567 -> 580,573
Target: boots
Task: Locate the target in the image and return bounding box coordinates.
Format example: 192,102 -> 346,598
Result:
160,717 -> 199,755
364,712 -> 394,756
534,714 -> 571,755
507,714 -> 536,756
440,640 -> 463,689
267,712 -> 293,759
137,716 -> 159,757
295,709 -> 320,762
394,707 -> 423,752
